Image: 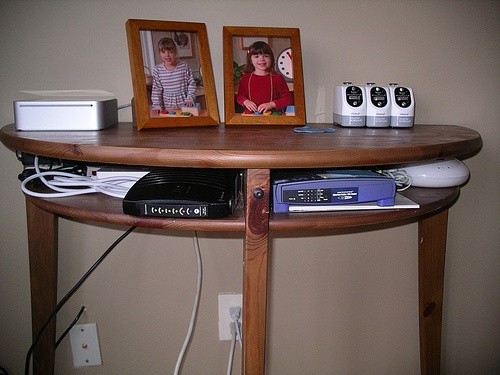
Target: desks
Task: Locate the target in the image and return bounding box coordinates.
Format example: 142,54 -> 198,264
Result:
0,122 -> 484,375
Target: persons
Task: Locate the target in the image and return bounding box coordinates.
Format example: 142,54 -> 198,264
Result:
152,37 -> 197,114
236,41 -> 293,115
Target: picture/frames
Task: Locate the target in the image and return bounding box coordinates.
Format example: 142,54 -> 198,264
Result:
223,26 -> 306,127
125,19 -> 221,131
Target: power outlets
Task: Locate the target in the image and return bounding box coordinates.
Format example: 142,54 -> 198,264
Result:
218,294 -> 242,341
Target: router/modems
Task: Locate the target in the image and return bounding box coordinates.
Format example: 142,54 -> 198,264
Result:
271,168 -> 397,213
123,169 -> 243,219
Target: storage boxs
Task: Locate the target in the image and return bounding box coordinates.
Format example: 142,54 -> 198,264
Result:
13,98 -> 118,131
